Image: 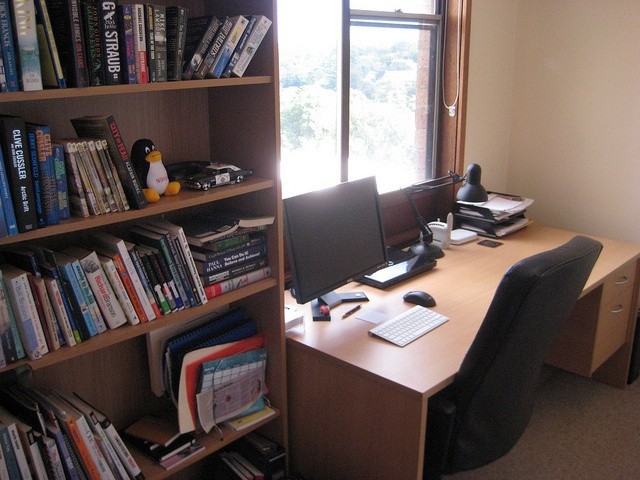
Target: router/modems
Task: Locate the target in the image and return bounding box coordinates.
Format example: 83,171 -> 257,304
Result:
451,229 -> 478,246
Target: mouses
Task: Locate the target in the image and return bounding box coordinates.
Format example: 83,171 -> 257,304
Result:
403,290 -> 436,307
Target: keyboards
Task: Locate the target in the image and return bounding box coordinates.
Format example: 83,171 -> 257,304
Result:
368,305 -> 449,348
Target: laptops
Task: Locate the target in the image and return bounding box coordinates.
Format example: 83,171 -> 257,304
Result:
354,244 -> 438,289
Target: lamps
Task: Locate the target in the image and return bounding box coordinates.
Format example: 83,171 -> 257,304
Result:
401,162 -> 489,261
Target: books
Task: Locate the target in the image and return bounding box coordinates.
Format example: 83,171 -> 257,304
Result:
1,219 -> 210,371
152,4 -> 167,82
193,15 -> 234,80
148,305 -> 280,429
34,0 -> 68,89
98,1 -> 125,86
10,0 -> 44,92
222,14 -> 259,78
132,4 -> 150,83
0,0 -> 21,91
166,208 -> 277,300
202,434 -> 286,480
0,115 -> 147,237
166,6 -> 190,81
0,42 -> 8,93
0,386 -> 199,480
118,5 -> 138,84
183,15 -> 222,80
144,4 -> 158,82
46,1 -> 91,88
232,14 -> 274,78
208,15 -> 250,79
81,1 -> 106,86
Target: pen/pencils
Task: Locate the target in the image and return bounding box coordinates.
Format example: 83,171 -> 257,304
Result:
342,305 -> 362,317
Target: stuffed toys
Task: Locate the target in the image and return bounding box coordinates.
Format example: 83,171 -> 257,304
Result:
129,137 -> 181,203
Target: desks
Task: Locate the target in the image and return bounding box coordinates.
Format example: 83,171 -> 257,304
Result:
286,217 -> 637,480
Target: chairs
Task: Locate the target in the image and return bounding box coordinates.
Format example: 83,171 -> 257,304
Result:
426,232 -> 604,478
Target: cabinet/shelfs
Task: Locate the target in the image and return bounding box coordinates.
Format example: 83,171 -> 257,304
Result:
0,0 -> 292,480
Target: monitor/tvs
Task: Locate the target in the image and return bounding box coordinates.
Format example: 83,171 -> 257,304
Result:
282,176 -> 390,322
